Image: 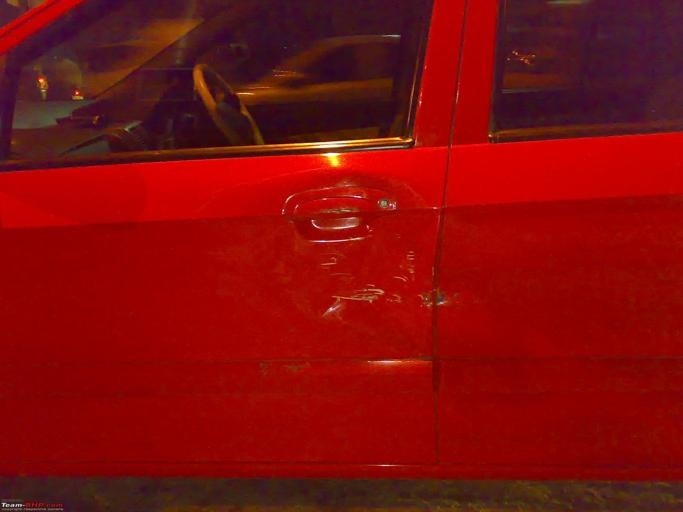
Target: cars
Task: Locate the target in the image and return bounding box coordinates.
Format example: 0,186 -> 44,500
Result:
1,0 -> 683,481
215,33 -> 563,108
